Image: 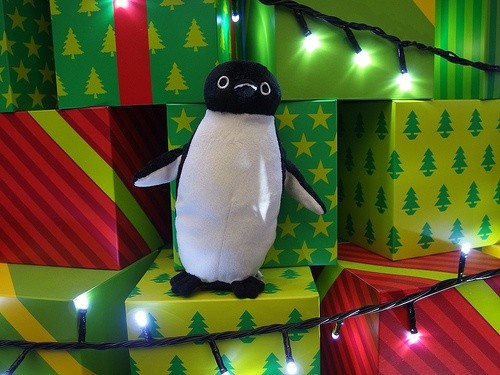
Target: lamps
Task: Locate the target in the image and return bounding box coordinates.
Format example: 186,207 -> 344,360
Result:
256,0 -> 500,92
0,240 -> 499,375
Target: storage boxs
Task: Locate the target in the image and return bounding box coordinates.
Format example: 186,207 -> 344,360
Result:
335,97 -> 500,263
0,264 -> 163,375
307,242 -> 500,375
0,0 -> 59,113
49,0 -> 232,110
0,104 -> 167,271
163,95 -> 338,269
239,0 -> 437,100
123,246 -> 322,375
